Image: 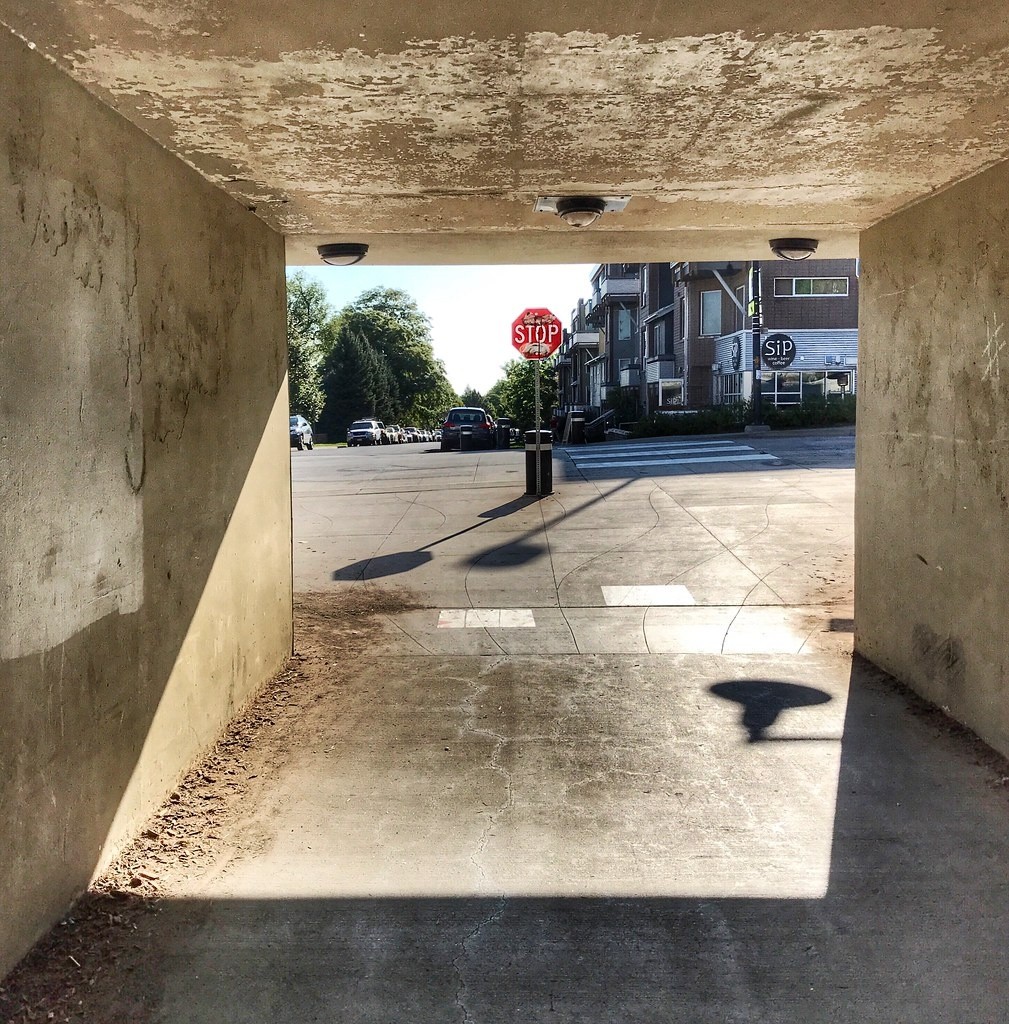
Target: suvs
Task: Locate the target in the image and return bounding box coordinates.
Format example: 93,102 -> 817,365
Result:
290,415 -> 313,451
347,420 -> 381,447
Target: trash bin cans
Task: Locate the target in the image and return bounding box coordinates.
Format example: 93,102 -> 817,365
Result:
570,410 -> 586,445
496,418 -> 511,449
460,425 -> 474,451
525,430 -> 553,494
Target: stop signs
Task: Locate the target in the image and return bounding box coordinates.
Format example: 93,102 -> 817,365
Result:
512,308 -> 563,360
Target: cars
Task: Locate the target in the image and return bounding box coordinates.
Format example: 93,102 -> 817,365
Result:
441,408 -> 519,452
374,419 -> 442,444
